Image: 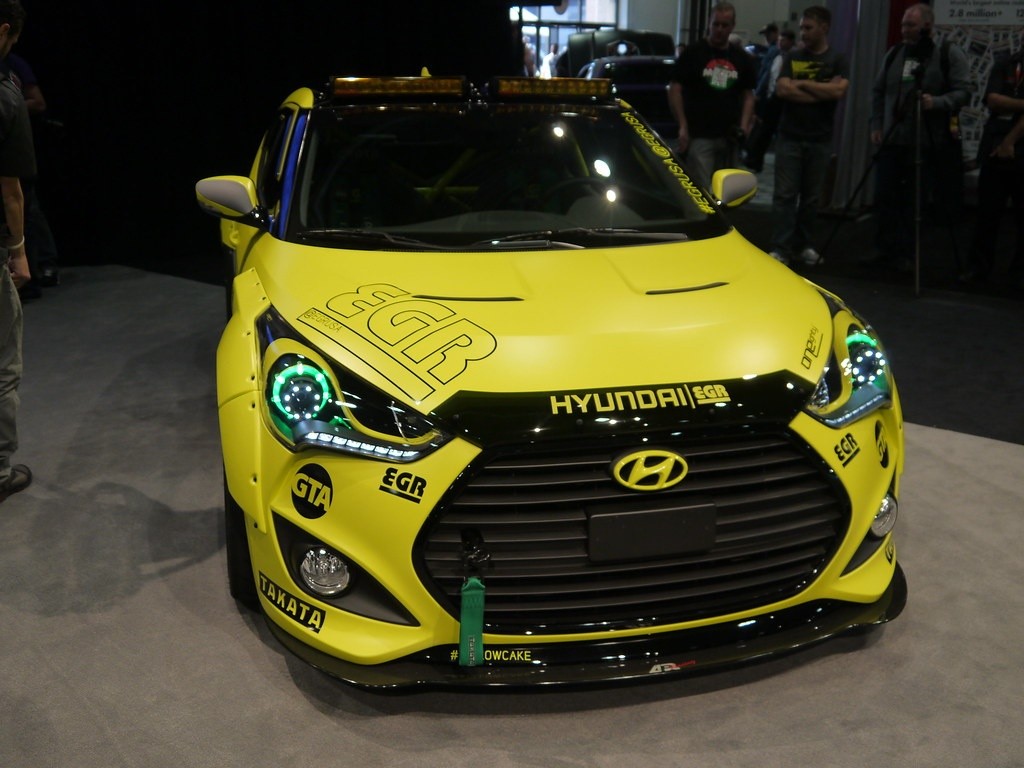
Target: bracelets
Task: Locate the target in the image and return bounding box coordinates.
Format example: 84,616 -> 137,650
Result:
7,234 -> 25,249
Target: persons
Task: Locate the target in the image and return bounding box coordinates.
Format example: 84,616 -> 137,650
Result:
728,32 -> 742,47
543,43 -> 580,92
768,5 -> 852,265
678,43 -> 685,52
752,23 -> 780,113
0,0 -> 36,501
668,2 -> 759,190
0,52 -> 59,299
523,35 -> 534,78
743,29 -> 796,172
958,40 -> 1024,291
863,3 -> 972,271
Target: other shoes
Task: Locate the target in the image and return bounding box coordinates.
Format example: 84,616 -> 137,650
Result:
801,247 -> 824,266
0,464 -> 32,503
36,261 -> 67,288
18,282 -> 41,301
768,250 -> 781,261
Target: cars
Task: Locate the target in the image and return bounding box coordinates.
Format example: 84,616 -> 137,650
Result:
191,76 -> 904,699
578,56 -> 805,249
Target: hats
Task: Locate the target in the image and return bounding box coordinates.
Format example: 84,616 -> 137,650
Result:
759,24 -> 778,34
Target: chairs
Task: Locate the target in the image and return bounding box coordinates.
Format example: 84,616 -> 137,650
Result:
471,145 -> 578,215
325,161 -> 418,231
